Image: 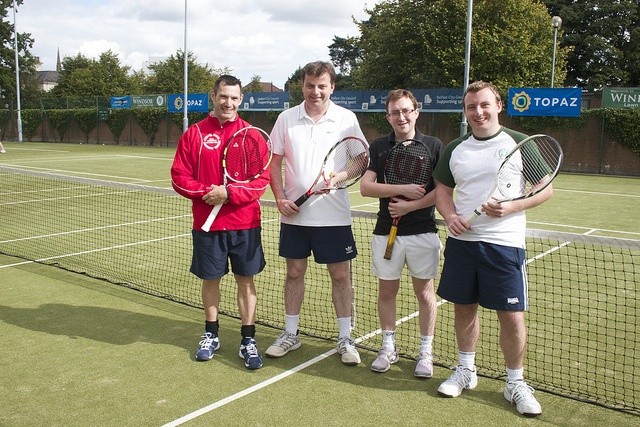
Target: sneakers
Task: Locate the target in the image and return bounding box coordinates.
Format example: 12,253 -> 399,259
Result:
238,337 -> 263,370
337,336 -> 362,365
371,344 -> 400,373
437,365 -> 478,398
414,352 -> 434,378
196,332 -> 221,362
503,378 -> 543,416
265,329 -> 302,358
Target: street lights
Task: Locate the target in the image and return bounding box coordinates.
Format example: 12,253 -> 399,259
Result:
550,15 -> 562,88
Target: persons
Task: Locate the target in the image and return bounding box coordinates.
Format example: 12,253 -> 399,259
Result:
432,80 -> 553,416
0,141 -> 6,153
170,75 -> 270,370
265,61 -> 371,364
360,89 -> 445,378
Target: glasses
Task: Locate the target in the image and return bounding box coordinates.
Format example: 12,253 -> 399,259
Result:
387,109 -> 415,117
